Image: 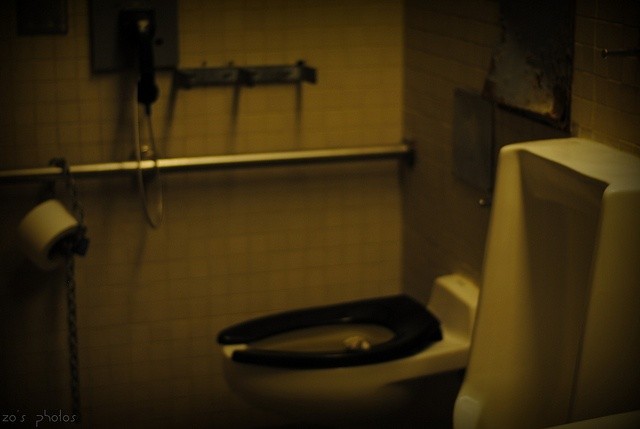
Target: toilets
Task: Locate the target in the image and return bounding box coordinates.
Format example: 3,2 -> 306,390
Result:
215,274 -> 479,418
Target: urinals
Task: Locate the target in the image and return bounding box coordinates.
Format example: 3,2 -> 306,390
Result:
458,126 -> 640,427
